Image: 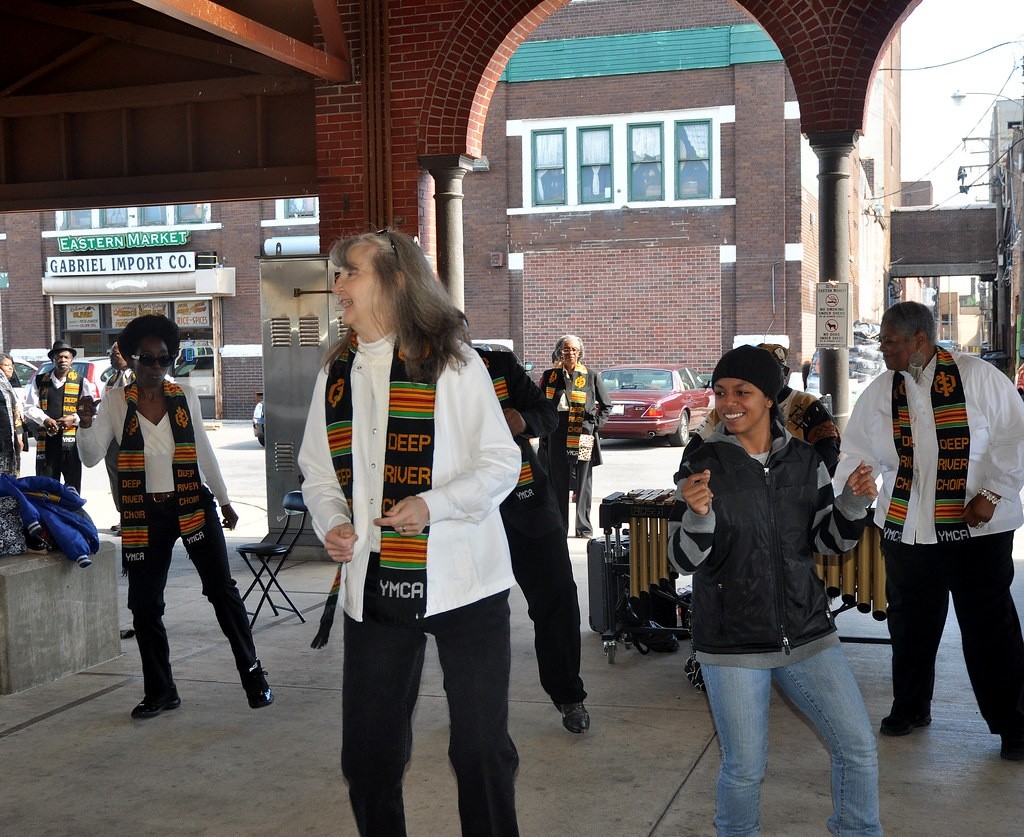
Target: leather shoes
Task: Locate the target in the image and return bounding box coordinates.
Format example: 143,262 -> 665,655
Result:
243,664 -> 273,709
561,701 -> 589,733
131,684 -> 180,718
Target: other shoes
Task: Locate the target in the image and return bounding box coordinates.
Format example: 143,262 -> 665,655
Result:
572,495 -> 579,503
880,710 -> 931,736
580,533 -> 593,539
116,528 -> 123,537
110,521 -> 122,530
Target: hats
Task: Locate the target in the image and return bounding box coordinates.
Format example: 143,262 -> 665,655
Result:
48,339 -> 78,358
711,345 -> 784,399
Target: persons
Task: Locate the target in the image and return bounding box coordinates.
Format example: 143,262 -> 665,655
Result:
297,227 -> 522,837
667,343 -> 884,837
76,314 -> 274,717
537,335 -> 614,538
468,348 -> 590,734
830,301 -> 1024,761
0,353 -> 29,479
23,340 -> 94,498
675,345 -> 841,695
101,341 -> 135,537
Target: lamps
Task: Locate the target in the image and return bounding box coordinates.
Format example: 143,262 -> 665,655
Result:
472,155 -> 489,171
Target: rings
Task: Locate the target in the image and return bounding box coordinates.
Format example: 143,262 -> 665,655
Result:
402,526 -> 406,532
79,405 -> 84,412
976,522 -> 983,529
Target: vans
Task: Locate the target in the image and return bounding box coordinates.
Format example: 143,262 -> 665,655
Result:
10,357 -> 112,436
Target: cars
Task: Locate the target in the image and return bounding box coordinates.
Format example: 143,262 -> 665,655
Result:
940,339 -> 954,353
470,343 -> 534,372
597,364 -> 715,447
252,395 -> 264,446
1015,343 -> 1024,400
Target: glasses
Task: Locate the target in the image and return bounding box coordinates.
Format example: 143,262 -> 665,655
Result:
376,229 -> 399,265
128,352 -> 176,367
560,348 -> 580,355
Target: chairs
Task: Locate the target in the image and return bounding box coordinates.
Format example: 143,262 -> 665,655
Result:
236,491 -> 308,631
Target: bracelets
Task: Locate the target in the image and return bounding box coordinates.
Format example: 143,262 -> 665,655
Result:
978,489 -> 1000,505
